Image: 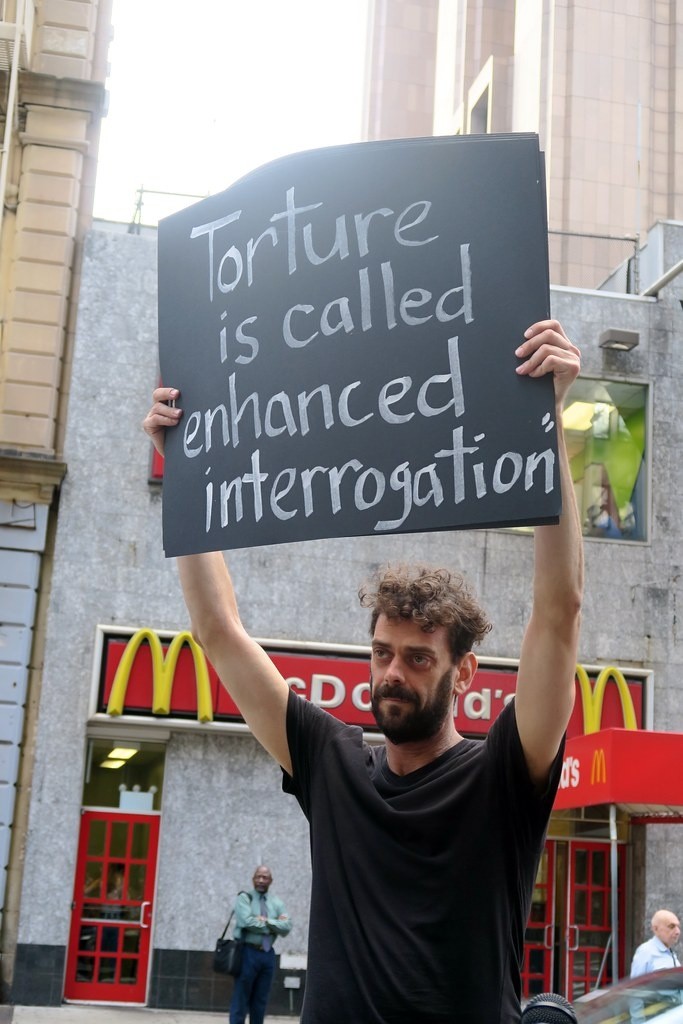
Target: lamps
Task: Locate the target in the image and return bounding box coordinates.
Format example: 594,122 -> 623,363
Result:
597,329 -> 638,351
106,740 -> 139,759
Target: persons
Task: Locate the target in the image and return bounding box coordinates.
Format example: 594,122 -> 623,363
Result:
229,865 -> 293,1024
629,909 -> 683,1024
144,320 -> 584,1024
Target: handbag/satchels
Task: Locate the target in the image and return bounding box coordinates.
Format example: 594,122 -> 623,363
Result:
211,938 -> 242,976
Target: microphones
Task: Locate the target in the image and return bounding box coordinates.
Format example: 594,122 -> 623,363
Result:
519,993 -> 578,1024
